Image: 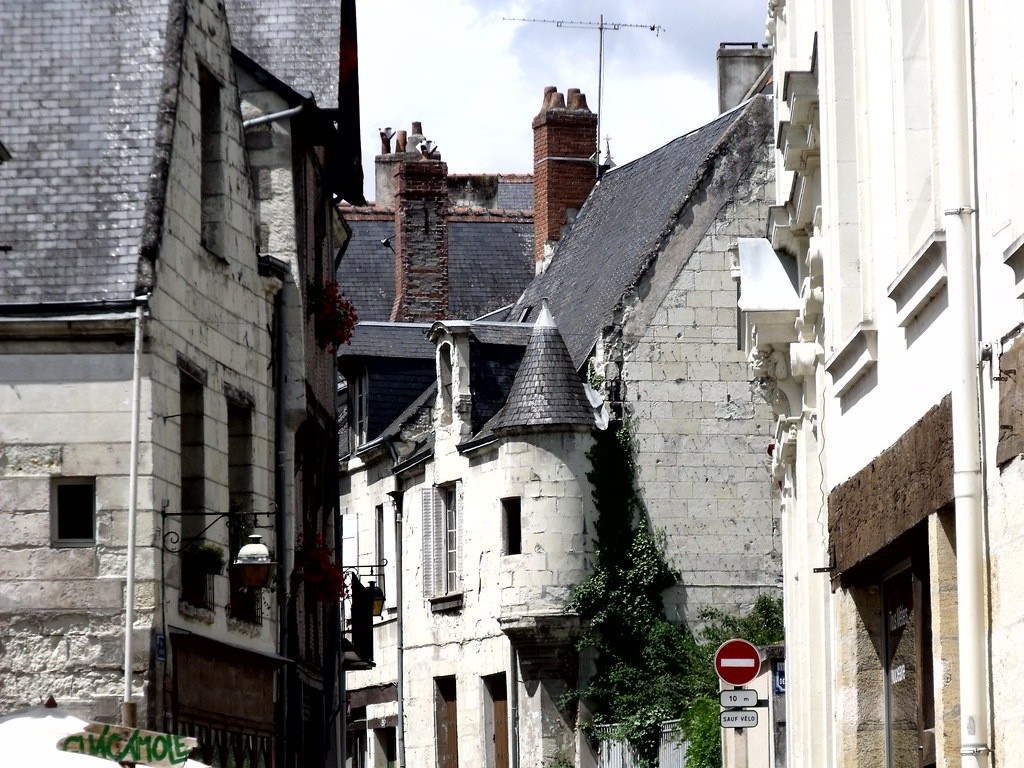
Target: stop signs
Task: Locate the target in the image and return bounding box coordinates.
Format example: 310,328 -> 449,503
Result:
714,637 -> 762,687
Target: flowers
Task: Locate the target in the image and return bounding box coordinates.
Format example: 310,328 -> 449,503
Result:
305,277 -> 356,352
291,530 -> 352,610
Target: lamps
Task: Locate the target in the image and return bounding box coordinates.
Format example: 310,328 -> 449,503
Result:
332,560 -> 386,619
160,501 -> 286,597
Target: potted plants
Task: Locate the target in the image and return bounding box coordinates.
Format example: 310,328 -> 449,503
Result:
195,542 -> 223,578
